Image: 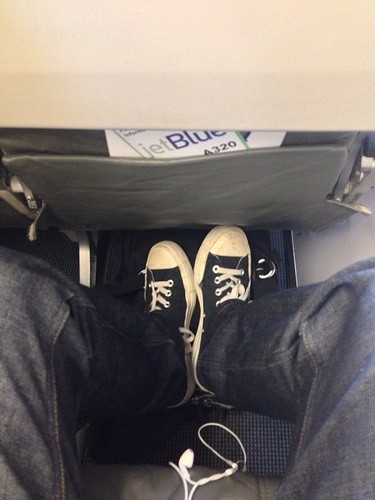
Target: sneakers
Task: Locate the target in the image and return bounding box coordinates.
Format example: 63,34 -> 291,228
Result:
142,240 -> 196,409
191,226 -> 253,394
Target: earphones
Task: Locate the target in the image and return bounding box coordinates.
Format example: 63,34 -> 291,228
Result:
178,448 -> 195,481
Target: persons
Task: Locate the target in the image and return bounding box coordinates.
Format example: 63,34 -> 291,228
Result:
0,223 -> 375,500
